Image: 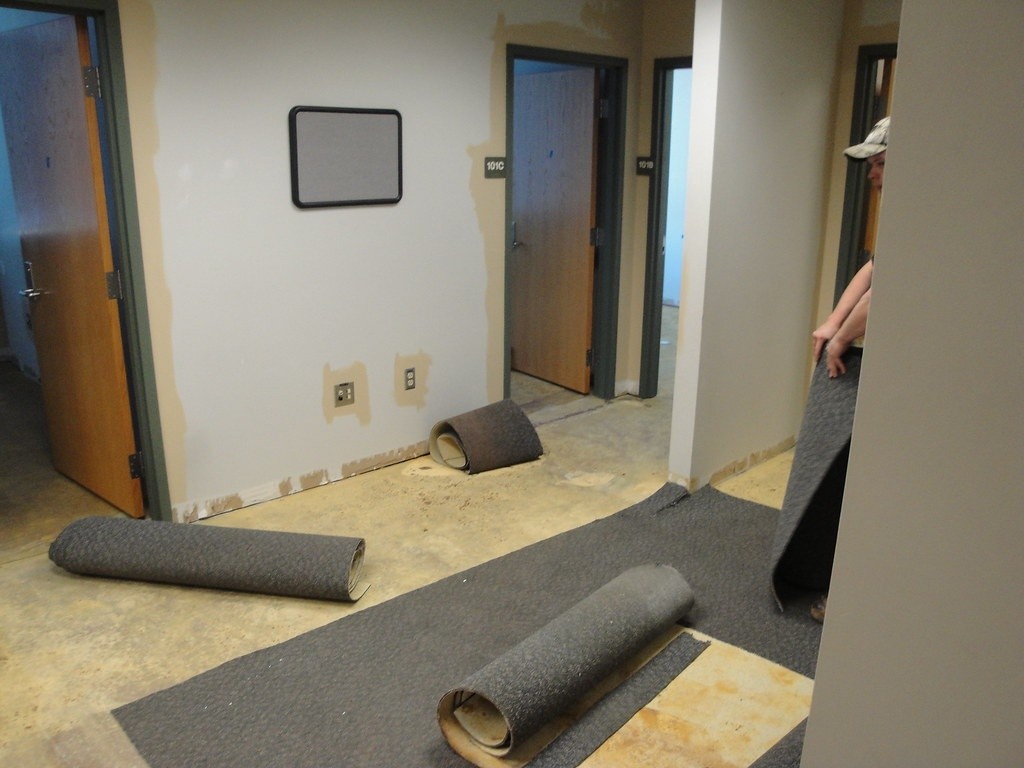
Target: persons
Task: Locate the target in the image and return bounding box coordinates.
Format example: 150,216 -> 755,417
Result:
810,117 -> 890,620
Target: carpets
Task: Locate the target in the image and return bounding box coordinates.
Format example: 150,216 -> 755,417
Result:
109,482 -> 823,768
766,341 -> 862,618
46,514 -> 372,606
430,398 -> 545,476
435,561 -> 699,768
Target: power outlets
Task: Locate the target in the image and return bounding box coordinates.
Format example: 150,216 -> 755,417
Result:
334,381 -> 354,408
405,367 -> 416,391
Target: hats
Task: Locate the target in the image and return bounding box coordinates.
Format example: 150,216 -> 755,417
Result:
842,116 -> 890,161
